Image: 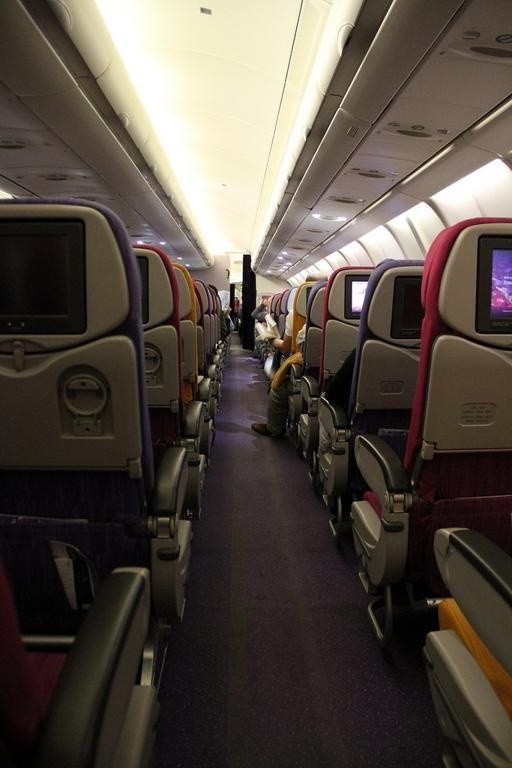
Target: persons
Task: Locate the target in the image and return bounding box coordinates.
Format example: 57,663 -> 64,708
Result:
209,283 -> 311,437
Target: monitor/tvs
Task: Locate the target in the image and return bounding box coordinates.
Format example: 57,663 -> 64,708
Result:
391,277 -> 424,339
0,222 -> 85,334
477,238 -> 512,334
345,275 -> 370,319
136,257 -> 148,323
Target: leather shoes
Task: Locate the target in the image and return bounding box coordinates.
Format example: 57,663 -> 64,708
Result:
252,423 -> 286,436
